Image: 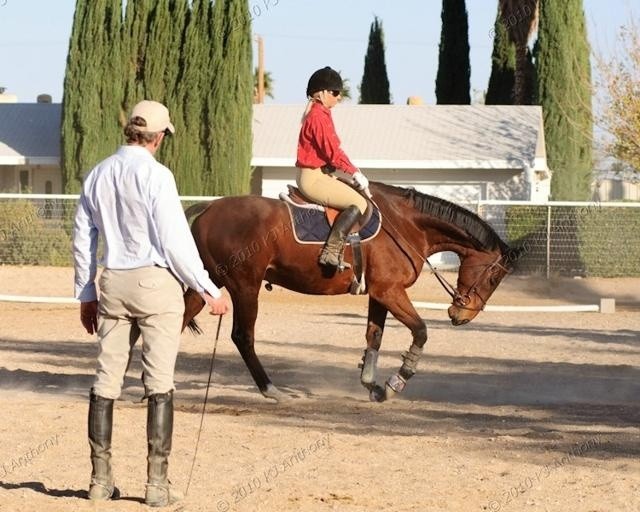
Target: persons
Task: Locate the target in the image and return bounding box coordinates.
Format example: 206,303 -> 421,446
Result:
72,100 -> 228,508
295,66 -> 372,269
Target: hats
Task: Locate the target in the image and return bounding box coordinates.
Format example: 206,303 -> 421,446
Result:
128,100 -> 176,134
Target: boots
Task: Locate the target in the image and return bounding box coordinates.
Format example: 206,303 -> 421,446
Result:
143,391 -> 178,508
317,204 -> 362,269
87,388 -> 124,502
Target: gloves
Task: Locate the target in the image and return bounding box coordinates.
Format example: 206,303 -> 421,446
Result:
351,171 -> 371,192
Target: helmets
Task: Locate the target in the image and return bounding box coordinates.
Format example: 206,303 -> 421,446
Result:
306,66 -> 344,96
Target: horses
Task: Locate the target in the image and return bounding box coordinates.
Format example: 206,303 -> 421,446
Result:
141,180 -> 524,408
